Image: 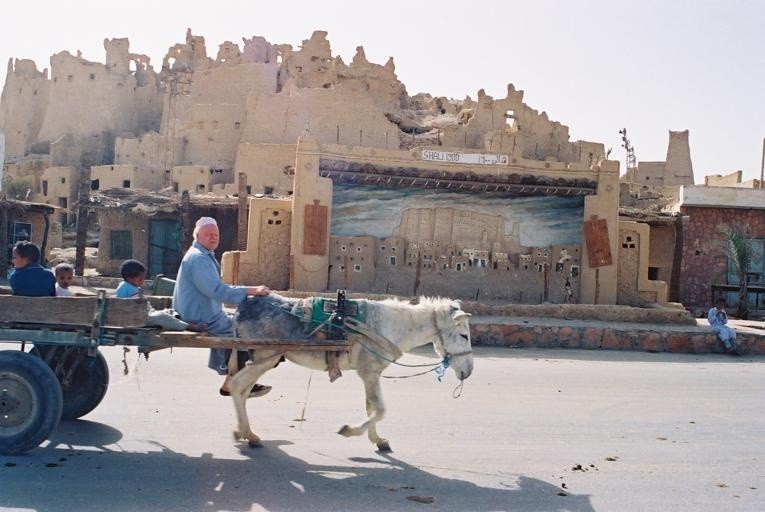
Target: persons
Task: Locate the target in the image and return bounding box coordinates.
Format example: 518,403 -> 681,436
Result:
8,239 -> 57,296
169,216 -> 273,399
52,262 -> 75,297
707,297 -> 739,354
113,259 -> 208,334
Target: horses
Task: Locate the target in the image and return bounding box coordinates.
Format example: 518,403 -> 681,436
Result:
227,292 -> 474,453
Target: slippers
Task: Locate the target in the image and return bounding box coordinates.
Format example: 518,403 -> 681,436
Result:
248,384 -> 272,397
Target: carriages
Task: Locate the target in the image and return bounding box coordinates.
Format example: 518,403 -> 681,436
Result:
0,275 -> 475,458
